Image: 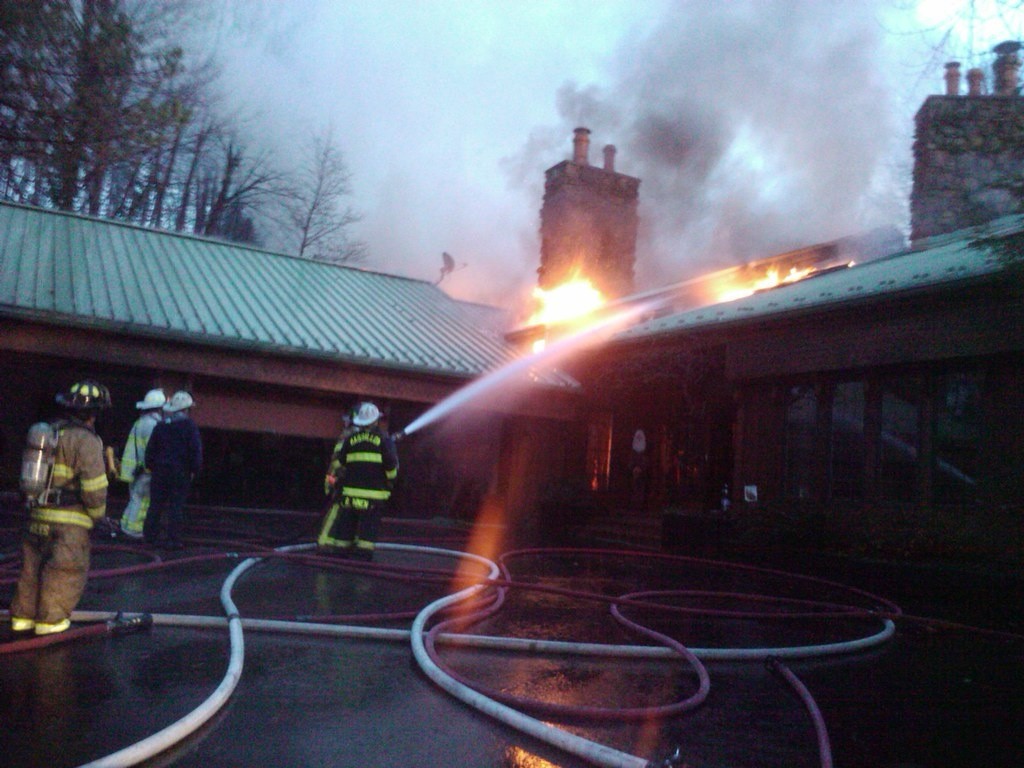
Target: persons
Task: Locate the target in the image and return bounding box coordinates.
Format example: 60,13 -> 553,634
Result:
317,402 -> 398,559
8,380 -> 111,640
141,391 -> 202,551
118,389 -> 167,542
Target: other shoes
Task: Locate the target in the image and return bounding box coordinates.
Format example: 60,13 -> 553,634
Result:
166,542 -> 182,549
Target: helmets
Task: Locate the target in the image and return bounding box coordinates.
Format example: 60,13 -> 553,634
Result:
135,390 -> 165,410
162,391 -> 192,413
353,401 -> 379,427
64,378 -> 111,409
